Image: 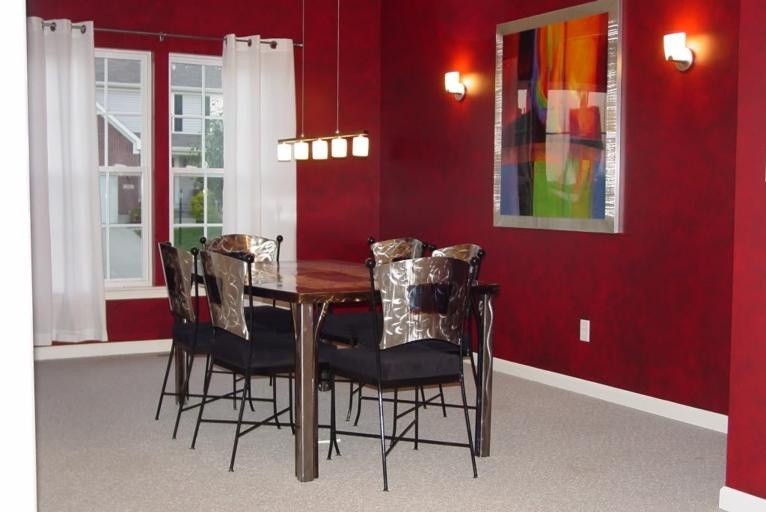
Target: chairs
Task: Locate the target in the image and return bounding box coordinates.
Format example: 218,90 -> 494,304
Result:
156,240 -> 281,441
200,232 -> 285,263
367,233 -> 485,278
190,247 -> 339,473
319,255 -> 481,491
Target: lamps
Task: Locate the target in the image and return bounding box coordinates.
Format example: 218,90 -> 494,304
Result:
276,0 -> 369,161
445,72 -> 465,101
663,32 -> 693,72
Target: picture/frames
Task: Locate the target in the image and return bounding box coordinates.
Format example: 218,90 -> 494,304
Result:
492,0 -> 624,233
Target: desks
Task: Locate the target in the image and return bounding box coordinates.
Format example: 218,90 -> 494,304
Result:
175,259 -> 500,482
